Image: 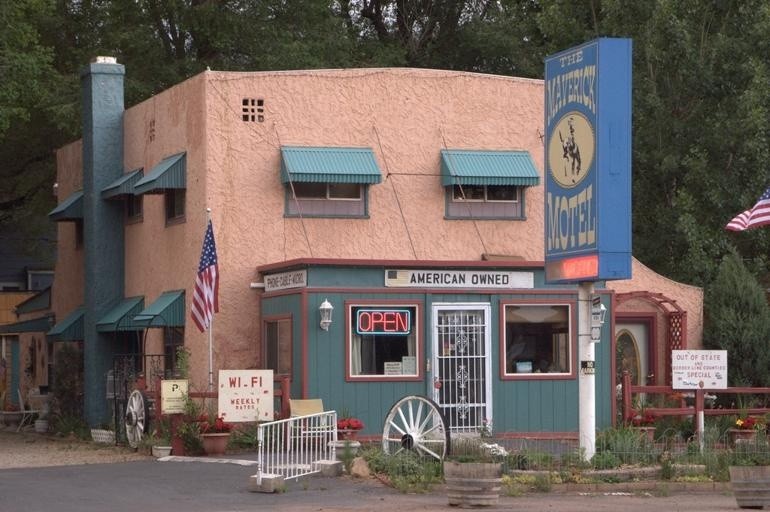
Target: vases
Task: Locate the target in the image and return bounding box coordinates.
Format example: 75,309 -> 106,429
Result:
337,430 -> 359,440
199,432 -> 231,458
730,429 -> 757,446
640,427 -> 655,445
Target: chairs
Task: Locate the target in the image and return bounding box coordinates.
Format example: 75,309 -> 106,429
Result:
16,389 -> 50,433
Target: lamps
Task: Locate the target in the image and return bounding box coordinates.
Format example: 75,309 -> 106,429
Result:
318,298 -> 334,331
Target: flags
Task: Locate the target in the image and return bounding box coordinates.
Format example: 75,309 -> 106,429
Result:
190,220 -> 219,333
724,188 -> 770,231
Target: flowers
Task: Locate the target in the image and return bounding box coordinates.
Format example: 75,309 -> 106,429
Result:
628,409 -> 656,427
735,416 -> 759,429
198,413 -> 237,433
336,408 -> 365,429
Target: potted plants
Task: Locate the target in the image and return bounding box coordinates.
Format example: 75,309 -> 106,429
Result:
728,454 -> 770,509
442,448 -> 505,506
150,434 -> 173,458
